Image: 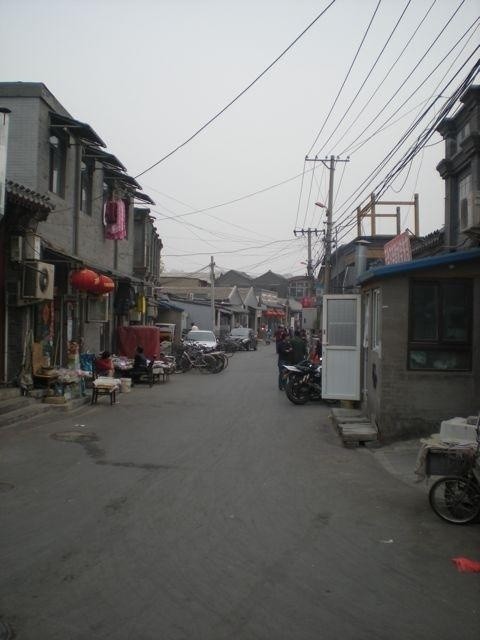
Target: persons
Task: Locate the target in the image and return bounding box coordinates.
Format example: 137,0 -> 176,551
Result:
191,322 -> 200,332
262,322 -> 322,391
94,351 -> 116,377
129,346 -> 148,386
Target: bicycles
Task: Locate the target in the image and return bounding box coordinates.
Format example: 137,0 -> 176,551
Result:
159,338 -> 229,375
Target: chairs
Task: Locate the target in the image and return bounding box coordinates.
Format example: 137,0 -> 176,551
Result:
133,359 -> 155,388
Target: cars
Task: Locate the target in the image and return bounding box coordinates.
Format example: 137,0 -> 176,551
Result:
181,330 -> 220,353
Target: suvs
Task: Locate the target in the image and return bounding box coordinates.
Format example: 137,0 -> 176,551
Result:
222,327 -> 258,351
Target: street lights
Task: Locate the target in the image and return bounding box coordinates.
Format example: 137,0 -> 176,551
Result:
314,202 -> 333,358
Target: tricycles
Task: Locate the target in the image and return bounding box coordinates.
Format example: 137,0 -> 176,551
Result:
415,436 -> 480,525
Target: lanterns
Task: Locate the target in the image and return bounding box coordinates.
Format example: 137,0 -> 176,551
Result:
265,311 -> 285,319
87,274 -> 115,295
69,268 -> 100,293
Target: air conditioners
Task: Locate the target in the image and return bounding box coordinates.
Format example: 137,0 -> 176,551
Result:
22,260 -> 56,301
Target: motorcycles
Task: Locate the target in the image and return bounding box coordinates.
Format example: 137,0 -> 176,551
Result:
281,357 -> 322,406
